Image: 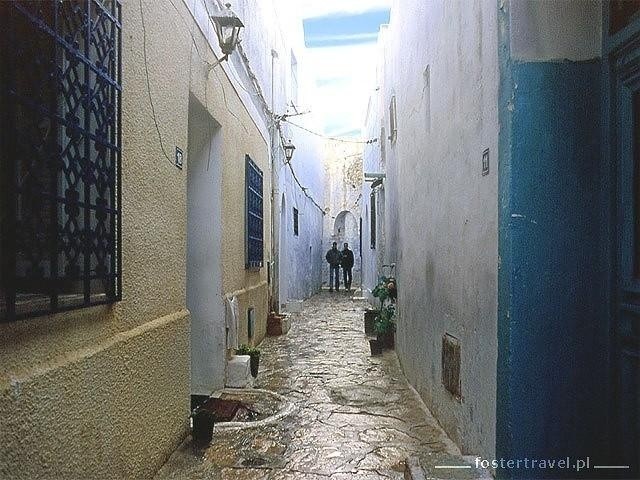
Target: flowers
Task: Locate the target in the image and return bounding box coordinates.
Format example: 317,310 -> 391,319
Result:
371,276 -> 397,337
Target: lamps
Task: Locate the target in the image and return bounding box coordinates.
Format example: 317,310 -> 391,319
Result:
283,139 -> 295,165
210,3 -> 246,75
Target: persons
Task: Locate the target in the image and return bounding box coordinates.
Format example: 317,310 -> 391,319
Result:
341,243 -> 354,292
326,242 -> 341,292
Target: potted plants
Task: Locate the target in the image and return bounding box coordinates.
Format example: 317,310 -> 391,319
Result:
190,401 -> 219,447
373,304 -> 397,349
236,344 -> 261,379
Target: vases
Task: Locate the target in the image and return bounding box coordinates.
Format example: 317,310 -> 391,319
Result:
369,339 -> 382,356
